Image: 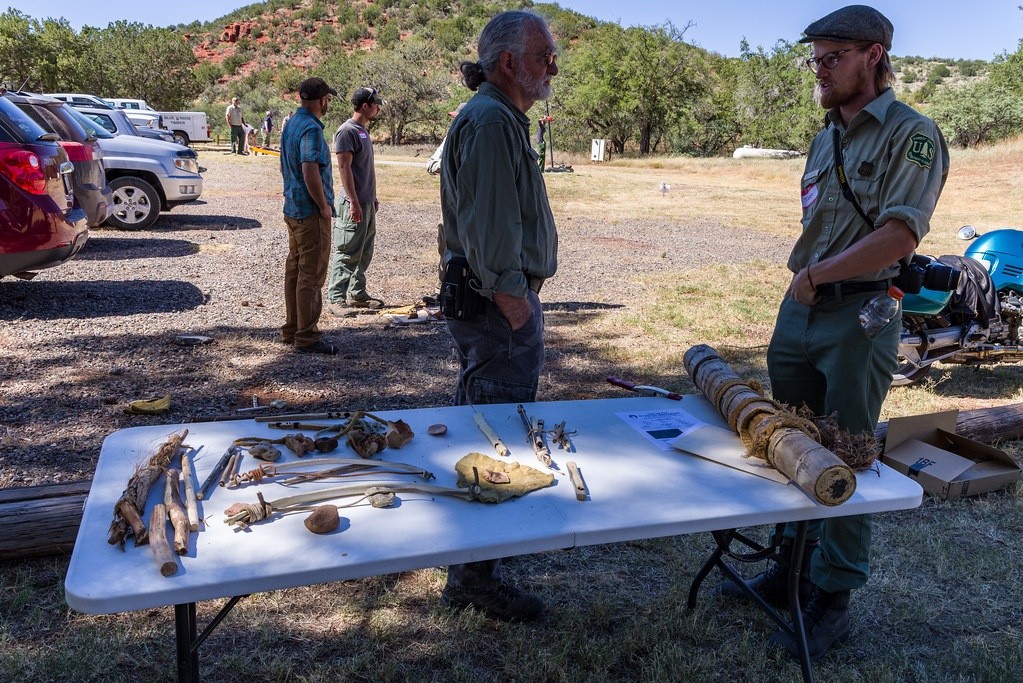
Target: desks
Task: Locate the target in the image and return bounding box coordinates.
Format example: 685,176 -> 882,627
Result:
64,389 -> 924,682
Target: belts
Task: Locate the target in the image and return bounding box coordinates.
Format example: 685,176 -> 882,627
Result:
528,277 -> 545,293
822,280 -> 888,294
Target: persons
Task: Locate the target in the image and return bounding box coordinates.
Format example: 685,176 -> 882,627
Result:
421,102 -> 467,304
225,97 -> 247,154
537,115 -> 549,173
279,78 -> 337,353
281,110 -> 292,132
261,110 -> 273,147
439,10 -> 559,624
327,87 -> 383,317
718,7 -> 947,666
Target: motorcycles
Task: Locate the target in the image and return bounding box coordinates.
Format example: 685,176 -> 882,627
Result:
886,224 -> 1023,387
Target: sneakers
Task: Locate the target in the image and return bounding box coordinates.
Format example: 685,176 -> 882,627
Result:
294,340 -> 338,355
282,337 -> 295,343
329,301 -> 357,318
347,298 -> 384,309
441,572 -> 544,624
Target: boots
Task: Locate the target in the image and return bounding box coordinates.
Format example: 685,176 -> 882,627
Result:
711,541 -> 819,605
767,583 -> 851,661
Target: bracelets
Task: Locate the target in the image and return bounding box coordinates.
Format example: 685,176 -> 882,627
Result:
806,263 -> 815,292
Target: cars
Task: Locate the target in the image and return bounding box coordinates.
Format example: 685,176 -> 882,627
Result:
0,86 -> 93,278
8,89 -> 114,227
16,91 -> 207,231
46,93 -> 216,144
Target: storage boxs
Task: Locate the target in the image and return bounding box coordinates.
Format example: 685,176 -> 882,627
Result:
880,409 -> 1022,504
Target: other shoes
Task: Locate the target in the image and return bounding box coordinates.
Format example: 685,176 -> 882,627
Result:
237,151 -> 246,155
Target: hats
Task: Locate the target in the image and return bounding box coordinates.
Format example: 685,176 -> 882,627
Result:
351,89 -> 388,106
300,78 -> 337,99
800,5 -> 893,51
266,111 -> 273,115
448,103 -> 467,117
254,129 -> 259,136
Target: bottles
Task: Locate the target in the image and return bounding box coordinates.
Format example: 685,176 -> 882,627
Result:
859,286 -> 904,338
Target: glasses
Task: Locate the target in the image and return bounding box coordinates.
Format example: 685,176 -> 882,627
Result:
516,52 -> 558,64
806,45 -> 870,74
366,88 -> 377,102
